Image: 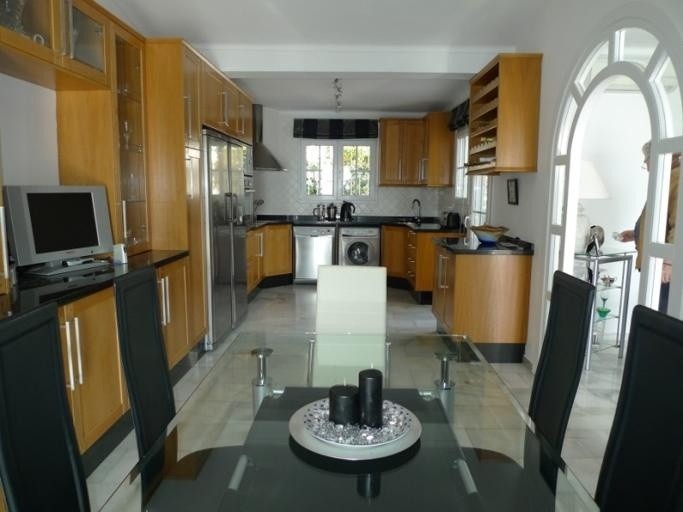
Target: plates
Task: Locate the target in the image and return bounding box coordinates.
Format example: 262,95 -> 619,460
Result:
288,398 -> 422,463
302,398 -> 411,447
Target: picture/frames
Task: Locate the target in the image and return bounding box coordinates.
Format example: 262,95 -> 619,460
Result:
505,179 -> 518,206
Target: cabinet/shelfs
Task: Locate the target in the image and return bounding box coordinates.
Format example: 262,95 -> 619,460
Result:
143,35 -> 204,369
52,283 -> 128,454
197,56 -> 254,145
375,116 -> 425,187
379,226 -> 406,279
265,227 -> 291,278
246,222 -> 264,294
430,239 -> 532,363
122,254 -> 189,410
423,110 -> 453,190
54,1 -> 151,257
574,250 -> 633,370
0,2 -> 113,91
465,52 -> 544,177
405,226 -> 463,305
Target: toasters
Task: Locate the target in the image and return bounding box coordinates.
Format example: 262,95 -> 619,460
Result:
439,211 -> 459,229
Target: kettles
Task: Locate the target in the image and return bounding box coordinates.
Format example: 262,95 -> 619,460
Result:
339,201 -> 355,221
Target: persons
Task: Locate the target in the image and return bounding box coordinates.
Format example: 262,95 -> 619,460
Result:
612,135 -> 682,314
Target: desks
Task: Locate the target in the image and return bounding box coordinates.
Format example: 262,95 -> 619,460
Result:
94,325 -> 604,511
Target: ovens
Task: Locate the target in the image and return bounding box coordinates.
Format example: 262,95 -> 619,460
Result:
291,227 -> 335,284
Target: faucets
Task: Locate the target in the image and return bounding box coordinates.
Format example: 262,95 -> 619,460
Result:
254,198 -> 264,224
410,198 -> 422,222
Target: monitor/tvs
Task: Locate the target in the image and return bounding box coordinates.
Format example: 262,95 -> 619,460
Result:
4,184 -> 114,276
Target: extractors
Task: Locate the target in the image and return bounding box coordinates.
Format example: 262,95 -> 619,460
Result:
252,104 -> 288,171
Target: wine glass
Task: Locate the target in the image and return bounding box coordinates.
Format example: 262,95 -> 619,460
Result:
600,291 -> 608,307
120,121 -> 134,152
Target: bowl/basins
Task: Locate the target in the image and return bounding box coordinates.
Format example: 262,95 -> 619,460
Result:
469,224 -> 507,244
595,307 -> 611,318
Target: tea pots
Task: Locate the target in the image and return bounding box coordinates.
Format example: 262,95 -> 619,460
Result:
312,204 -> 325,221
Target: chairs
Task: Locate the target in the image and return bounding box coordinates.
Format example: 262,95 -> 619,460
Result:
460,269 -> 597,512
112,262 -> 246,512
592,298 -> 681,511
0,297 -> 92,512
304,264 -> 390,387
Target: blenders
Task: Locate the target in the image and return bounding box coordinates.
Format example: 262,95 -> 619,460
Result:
326,203 -> 336,220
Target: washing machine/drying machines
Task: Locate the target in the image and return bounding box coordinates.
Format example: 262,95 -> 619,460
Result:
335,226 -> 381,266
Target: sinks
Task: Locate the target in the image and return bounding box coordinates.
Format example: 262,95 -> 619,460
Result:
408,221 -> 442,231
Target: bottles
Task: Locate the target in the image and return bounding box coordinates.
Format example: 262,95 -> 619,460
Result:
354,472 -> 383,498
327,384 -> 358,426
358,369 -> 382,431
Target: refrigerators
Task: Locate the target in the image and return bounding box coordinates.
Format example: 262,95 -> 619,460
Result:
201,126 -> 249,350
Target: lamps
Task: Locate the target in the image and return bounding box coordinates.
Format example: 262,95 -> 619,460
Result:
575,170 -> 608,217
